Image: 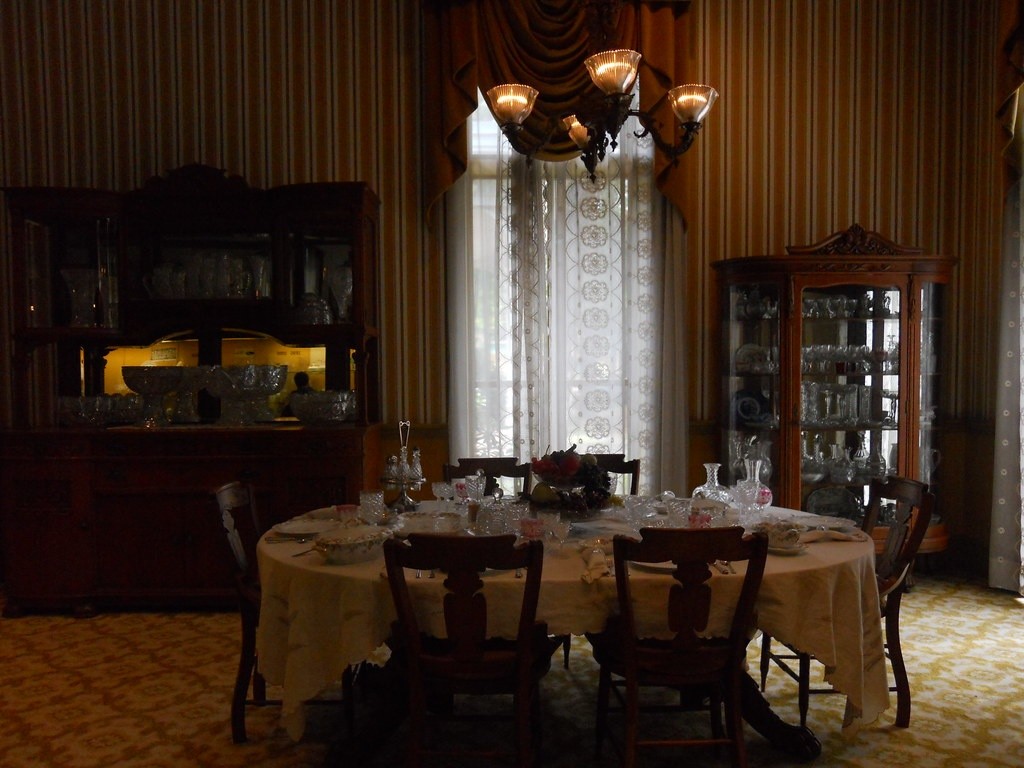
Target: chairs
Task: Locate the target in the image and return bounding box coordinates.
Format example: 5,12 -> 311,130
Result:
597,527 -> 769,768
581,454 -> 641,495
443,458 -> 533,495
210,479 -> 351,744
761,476 -> 937,728
384,531 -> 547,768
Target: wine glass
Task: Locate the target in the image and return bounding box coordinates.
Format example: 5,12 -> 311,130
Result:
801,298 -> 857,318
432,481 -> 454,510
122,367 -> 184,427
534,511 -> 569,555
457,483 -> 469,505
234,365 -> 288,422
800,344 -> 898,374
206,366 -> 246,424
162,366 -> 208,423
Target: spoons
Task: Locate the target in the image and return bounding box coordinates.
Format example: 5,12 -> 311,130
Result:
264,537 -> 311,543
291,545 -> 325,557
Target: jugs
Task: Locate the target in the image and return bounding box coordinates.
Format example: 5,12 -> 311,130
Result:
860,290 -> 890,318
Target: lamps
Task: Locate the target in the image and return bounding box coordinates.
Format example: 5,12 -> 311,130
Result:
486,0 -> 719,183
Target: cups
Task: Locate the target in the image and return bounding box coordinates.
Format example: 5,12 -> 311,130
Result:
294,291 -> 332,324
311,537 -> 364,565
474,488 -> 530,535
465,475 -> 486,504
359,489 -> 383,526
150,247 -> 271,298
56,393 -> 137,429
61,268 -> 101,329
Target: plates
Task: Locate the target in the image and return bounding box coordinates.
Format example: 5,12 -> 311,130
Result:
799,530 -> 825,543
735,343 -> 764,373
272,519 -> 340,534
397,512 -> 461,519
824,530 -> 869,542
765,542 -> 804,555
576,537 -> 613,554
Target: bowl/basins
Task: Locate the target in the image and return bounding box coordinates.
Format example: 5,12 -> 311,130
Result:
289,390 -> 356,425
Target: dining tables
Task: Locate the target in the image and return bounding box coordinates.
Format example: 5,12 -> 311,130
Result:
257,495 -> 891,767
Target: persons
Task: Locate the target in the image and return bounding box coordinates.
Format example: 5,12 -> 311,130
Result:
279,371 -> 318,418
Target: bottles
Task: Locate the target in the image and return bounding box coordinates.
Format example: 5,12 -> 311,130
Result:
96,217 -> 120,331
732,459 -> 773,509
331,270 -> 349,322
24,221 -> 53,329
802,431 -> 898,482
692,463 -> 732,506
801,380 -> 898,429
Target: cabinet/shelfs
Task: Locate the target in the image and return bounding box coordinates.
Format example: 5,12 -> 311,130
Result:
1,164 -> 380,614
710,224 -> 960,592
